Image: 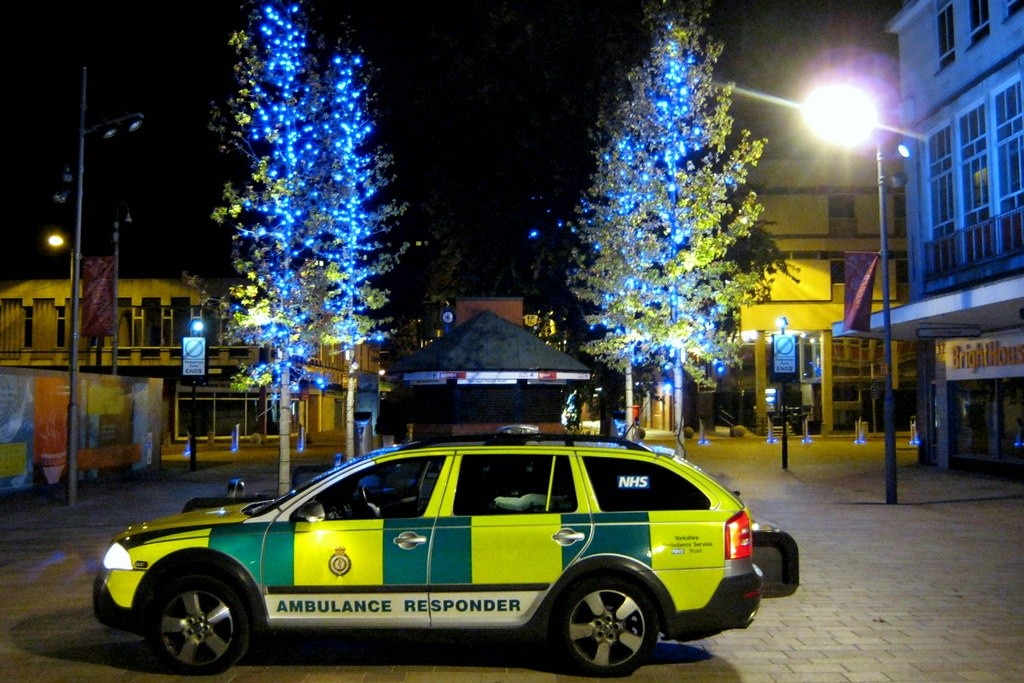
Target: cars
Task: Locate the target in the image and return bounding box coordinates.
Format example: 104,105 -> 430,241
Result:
180,430 -> 801,605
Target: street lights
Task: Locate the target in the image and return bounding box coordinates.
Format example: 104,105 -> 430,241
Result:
188,296 -> 222,471
798,52 -> 906,506
766,330 -> 790,469
65,66 -> 145,508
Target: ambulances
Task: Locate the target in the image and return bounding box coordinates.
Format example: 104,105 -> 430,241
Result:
92,445 -> 762,678
